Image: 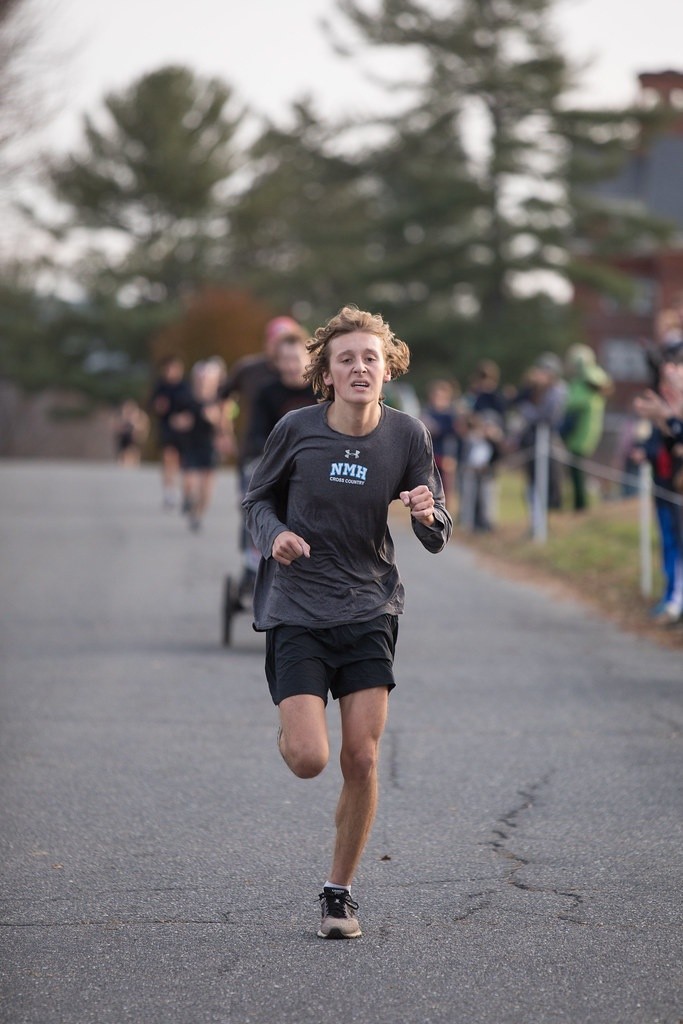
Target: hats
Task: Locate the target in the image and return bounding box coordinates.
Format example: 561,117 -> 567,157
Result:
266,316 -> 306,340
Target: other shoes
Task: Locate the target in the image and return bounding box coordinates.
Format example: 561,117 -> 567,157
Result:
164,498 -> 202,535
653,612 -> 676,628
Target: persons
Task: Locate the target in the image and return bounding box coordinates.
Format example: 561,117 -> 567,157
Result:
114,304 -> 683,941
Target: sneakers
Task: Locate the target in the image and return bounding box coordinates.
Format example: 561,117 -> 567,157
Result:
316,887 -> 362,938
